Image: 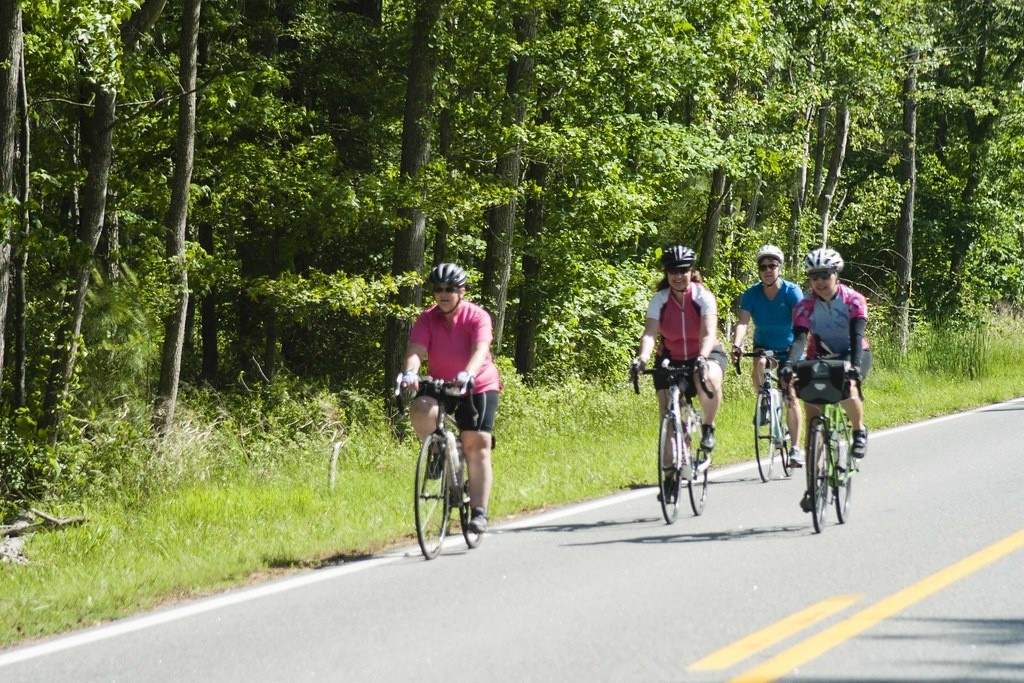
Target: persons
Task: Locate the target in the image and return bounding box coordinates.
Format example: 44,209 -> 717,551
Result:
783,248 -> 870,510
731,245 -> 803,468
630,246 -> 726,502
401,263 -> 501,534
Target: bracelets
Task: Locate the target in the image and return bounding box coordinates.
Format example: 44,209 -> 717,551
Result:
698,354 -> 707,361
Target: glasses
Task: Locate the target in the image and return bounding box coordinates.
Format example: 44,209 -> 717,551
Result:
809,270 -> 832,281
759,264 -> 779,271
433,286 -> 456,293
667,267 -> 690,275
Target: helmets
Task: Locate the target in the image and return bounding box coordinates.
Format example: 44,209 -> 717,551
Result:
661,245 -> 697,270
805,247 -> 844,274
756,244 -> 784,265
428,263 -> 467,288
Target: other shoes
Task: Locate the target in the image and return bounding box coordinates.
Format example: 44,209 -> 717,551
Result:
801,487 -> 819,509
428,459 -> 443,480
701,424 -> 716,452
657,478 -> 675,504
753,403 -> 769,426
789,448 -> 803,468
851,424 -> 868,458
468,506 -> 487,533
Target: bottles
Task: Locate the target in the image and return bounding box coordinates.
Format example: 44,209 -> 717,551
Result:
445,431 -> 464,481
673,420 -> 691,447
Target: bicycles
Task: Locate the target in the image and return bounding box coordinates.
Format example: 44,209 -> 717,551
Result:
730,343 -> 798,483
781,365 -> 867,534
630,355 -> 714,524
393,372 -> 496,561
